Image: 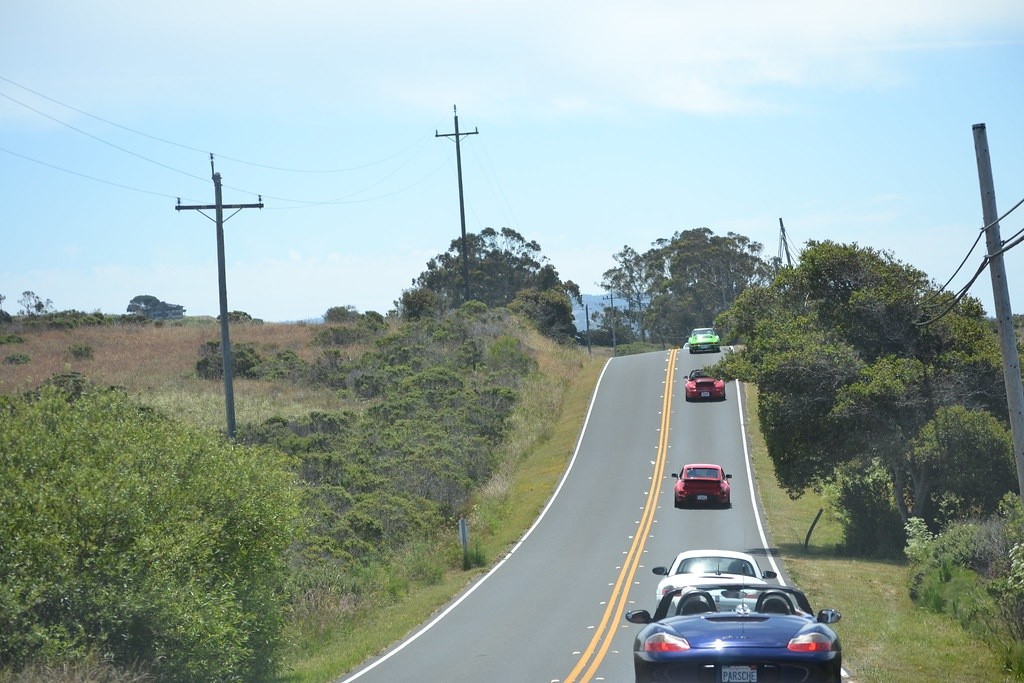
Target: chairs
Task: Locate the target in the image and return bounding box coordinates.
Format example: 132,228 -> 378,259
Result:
755,589 -> 796,615
675,591 -> 717,617
727,560 -> 749,576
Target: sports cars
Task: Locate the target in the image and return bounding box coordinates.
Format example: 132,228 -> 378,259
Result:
624,579 -> 844,683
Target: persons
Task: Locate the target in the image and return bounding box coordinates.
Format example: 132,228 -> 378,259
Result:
680,586 -> 710,614
728,561 -> 744,574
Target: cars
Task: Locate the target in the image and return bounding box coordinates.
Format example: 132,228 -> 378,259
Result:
683,368 -> 726,402
651,548 -> 778,618
688,327 -> 721,354
671,463 -> 732,509
